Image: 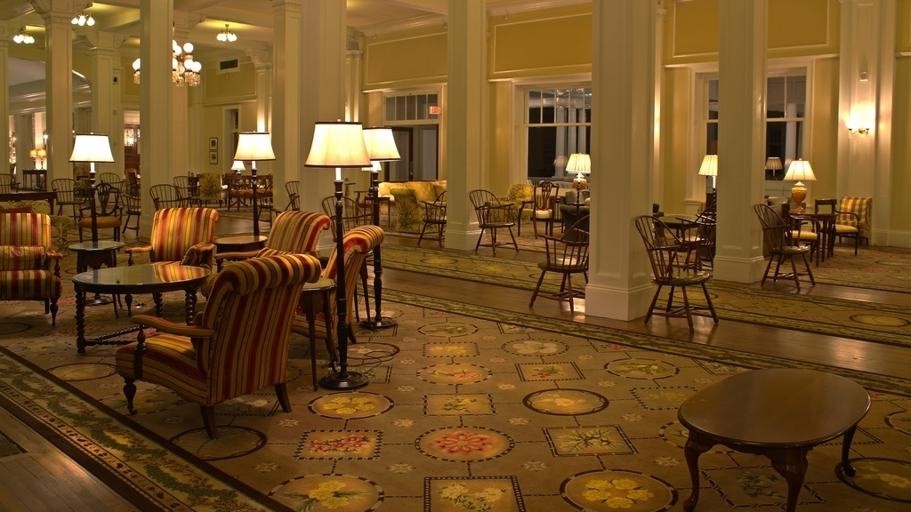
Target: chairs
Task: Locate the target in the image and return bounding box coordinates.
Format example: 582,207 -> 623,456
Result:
1,212 -> 63,328
214,211 -> 331,273
115,254 -> 322,439
303,224 -> 384,392
1,169 -> 141,252
125,209 -> 220,317
150,173 -> 299,228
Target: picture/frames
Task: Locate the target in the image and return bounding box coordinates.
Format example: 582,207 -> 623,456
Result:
209,137 -> 218,164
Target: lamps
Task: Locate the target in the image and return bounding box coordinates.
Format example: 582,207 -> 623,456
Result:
234,130 -> 275,238
784,160 -> 816,213
699,155 -> 719,191
131,21 -> 201,87
765,157 -> 782,176
10,30 -> 35,44
565,153 -> 590,178
68,14 -> 95,26
848,116 -> 870,137
303,123 -> 374,392
361,129 -> 400,329
68,133 -> 125,318
30,147 -> 46,169
210,27 -> 239,43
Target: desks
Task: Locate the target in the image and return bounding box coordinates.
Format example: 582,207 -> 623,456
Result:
300,278 -> 337,391
677,368 -> 870,511
